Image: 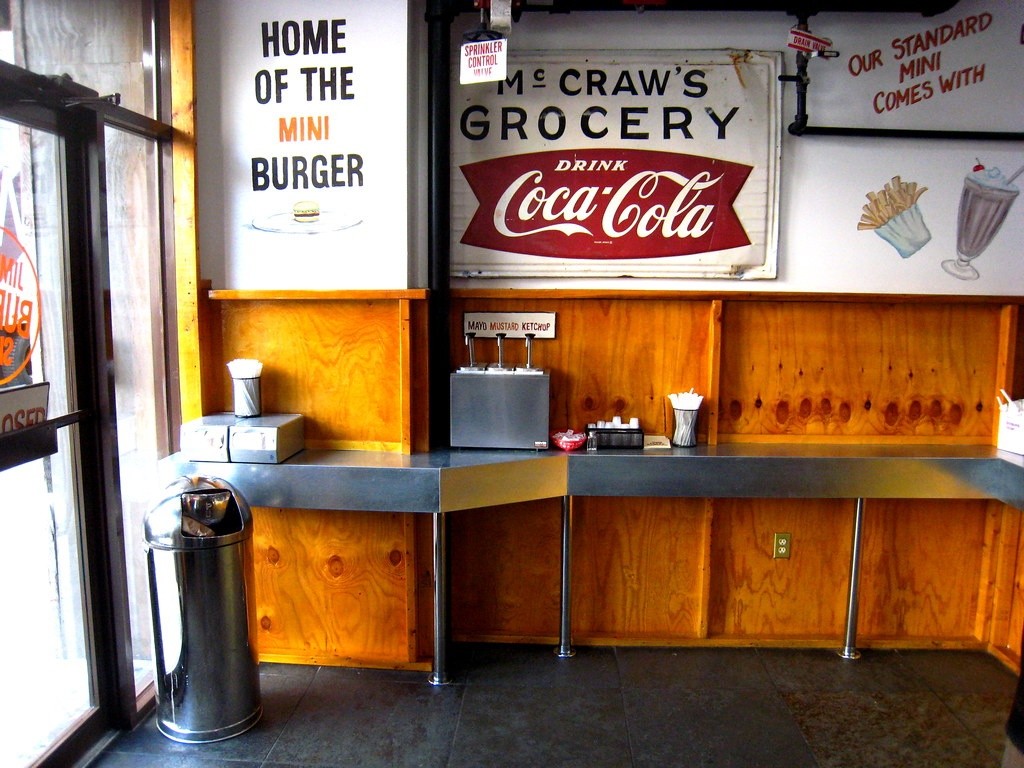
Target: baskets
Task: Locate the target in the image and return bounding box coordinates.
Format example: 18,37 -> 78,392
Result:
550,429 -> 587,450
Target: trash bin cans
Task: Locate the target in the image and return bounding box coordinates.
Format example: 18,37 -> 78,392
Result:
141,477 -> 264,745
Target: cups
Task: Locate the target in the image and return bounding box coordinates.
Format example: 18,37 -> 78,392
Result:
672,407 -> 697,446
941,177 -> 1020,280
231,378 -> 261,418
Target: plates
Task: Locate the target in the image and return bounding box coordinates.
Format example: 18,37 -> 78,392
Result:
253,212 -> 362,233
